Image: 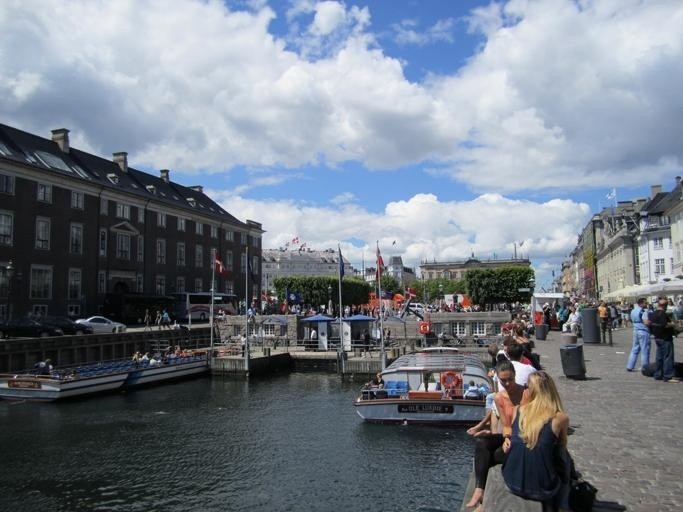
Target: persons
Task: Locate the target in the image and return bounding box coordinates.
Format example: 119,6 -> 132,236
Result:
144,309 -> 172,330
361,329 -> 373,358
438,331 -> 466,347
424,371 -> 432,391
462,302 -> 576,511
132,348 -> 194,367
426,302 -> 465,313
34,358 -> 54,378
360,373 -> 390,402
310,327 -> 317,352
239,296 -> 258,323
241,333 -> 250,357
377,326 -> 391,345
279,301 -> 387,318
563,293 -> 683,382
218,307 -> 227,324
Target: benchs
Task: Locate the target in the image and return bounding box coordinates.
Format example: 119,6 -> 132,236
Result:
481,464 -> 543,512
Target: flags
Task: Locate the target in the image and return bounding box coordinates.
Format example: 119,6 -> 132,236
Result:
215,253 -> 230,281
339,248 -> 345,279
375,248 -> 387,281
373,284 -> 417,300
247,251 -> 254,284
287,288 -> 302,305
605,188 -> 615,200
262,290 -> 273,304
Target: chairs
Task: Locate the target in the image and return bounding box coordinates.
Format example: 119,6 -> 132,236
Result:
384,381 -> 408,398
464,384 -> 488,394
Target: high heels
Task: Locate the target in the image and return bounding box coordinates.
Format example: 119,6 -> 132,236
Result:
471,486 -> 483,508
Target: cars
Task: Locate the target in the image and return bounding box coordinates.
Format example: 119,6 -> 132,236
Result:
1,315 -> 126,338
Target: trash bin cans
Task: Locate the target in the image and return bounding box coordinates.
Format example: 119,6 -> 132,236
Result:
582,308 -> 602,343
536,324 -> 549,340
560,344 -> 586,380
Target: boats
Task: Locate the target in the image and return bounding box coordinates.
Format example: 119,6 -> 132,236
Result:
0,345 -> 243,403
351,346 -> 496,427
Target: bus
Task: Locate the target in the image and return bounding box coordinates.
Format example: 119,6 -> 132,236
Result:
103,291 -> 239,326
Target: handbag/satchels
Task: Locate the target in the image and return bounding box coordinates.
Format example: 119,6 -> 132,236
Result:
569,482 -> 597,512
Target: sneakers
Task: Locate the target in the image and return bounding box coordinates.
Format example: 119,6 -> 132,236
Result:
642,368 -> 651,376
655,376 -> 679,382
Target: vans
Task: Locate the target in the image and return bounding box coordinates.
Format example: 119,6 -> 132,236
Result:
403,303 -> 438,315
